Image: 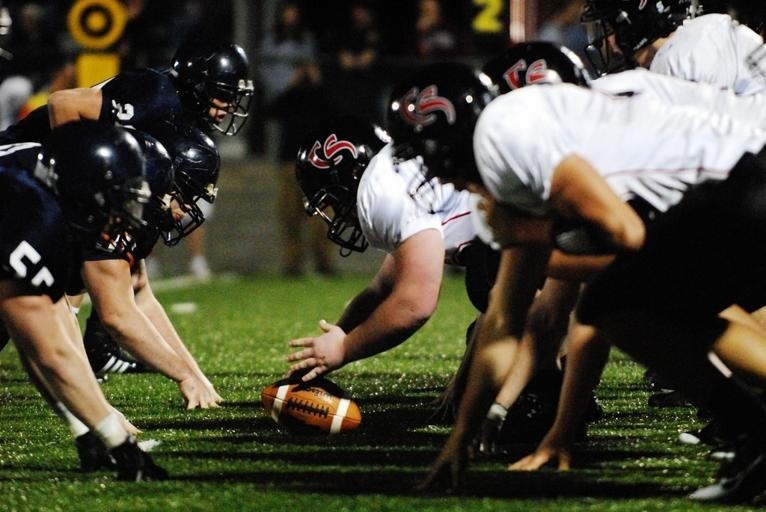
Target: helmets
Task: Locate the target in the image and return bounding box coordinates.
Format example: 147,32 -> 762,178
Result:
295,116 -> 392,255
119,127 -> 176,233
35,120 -> 167,264
157,124 -> 221,247
181,38 -> 255,136
578,0 -> 729,78
478,41 -> 590,109
381,63 -> 492,215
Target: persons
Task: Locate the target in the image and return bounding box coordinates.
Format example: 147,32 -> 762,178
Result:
3,2 -> 766,509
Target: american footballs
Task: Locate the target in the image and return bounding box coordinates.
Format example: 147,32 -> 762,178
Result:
261,376 -> 362,434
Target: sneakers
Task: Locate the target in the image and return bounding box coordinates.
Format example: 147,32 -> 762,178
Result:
87,347 -> 151,372
650,372 -> 765,505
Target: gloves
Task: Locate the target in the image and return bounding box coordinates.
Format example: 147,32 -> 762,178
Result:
57,401 -> 118,472
472,401 -> 508,442
93,409 -> 167,482
